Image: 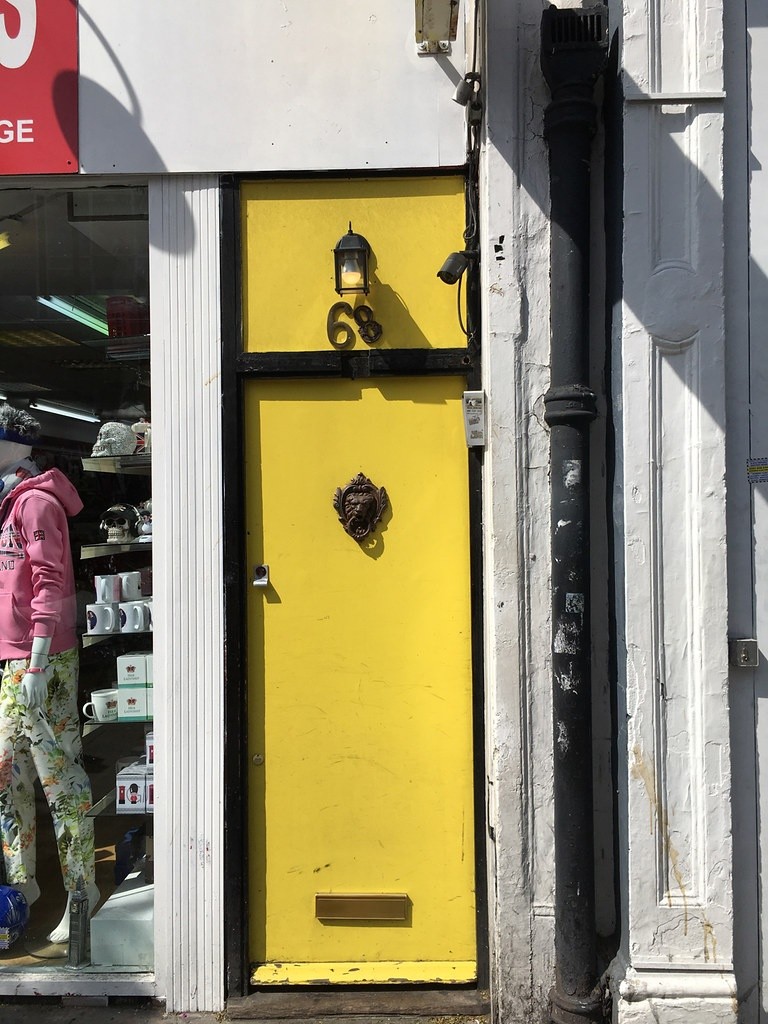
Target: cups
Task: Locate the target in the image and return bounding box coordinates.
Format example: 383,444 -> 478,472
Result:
116,757 -> 139,773
118,601 -> 144,631
118,571 -> 141,601
86,604 -> 115,633
94,575 -> 121,603
83,689 -> 117,721
136,597 -> 151,629
94,602 -> 118,630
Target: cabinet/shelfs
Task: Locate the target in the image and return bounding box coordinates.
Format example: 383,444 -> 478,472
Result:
72,450 -> 156,972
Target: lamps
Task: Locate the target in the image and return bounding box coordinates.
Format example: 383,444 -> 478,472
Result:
333,220 -> 372,296
0,294 -> 108,424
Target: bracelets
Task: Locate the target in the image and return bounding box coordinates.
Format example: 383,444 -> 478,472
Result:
24,667 -> 48,675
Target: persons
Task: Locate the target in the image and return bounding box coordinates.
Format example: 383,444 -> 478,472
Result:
0,402 -> 101,943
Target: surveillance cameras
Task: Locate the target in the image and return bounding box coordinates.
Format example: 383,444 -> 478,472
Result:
437,252 -> 469,285
